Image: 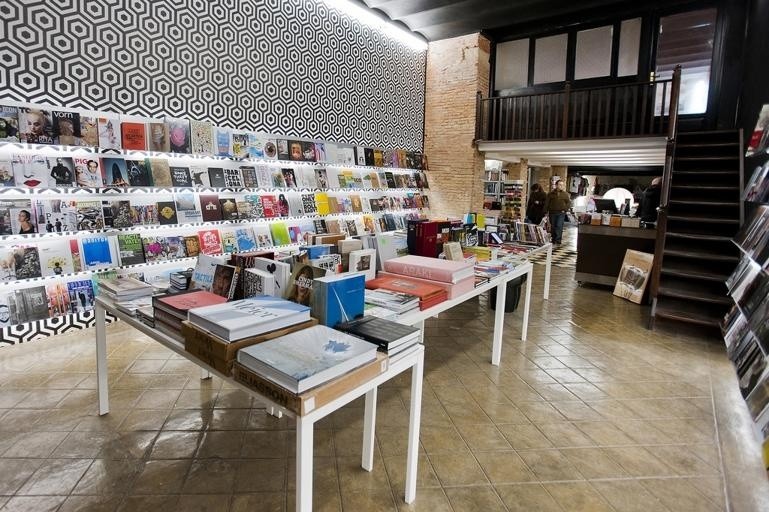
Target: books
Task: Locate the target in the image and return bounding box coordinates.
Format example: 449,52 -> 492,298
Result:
2,107 -> 145,324
300,211 -> 548,321
95,251 -> 422,393
121,120 -> 428,284
721,106 -> 769,446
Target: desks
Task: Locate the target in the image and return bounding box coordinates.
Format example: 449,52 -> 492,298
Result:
361,242 -> 552,471
94,277 -> 426,512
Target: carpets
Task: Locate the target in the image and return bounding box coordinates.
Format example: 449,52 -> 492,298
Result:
526,239 -> 577,269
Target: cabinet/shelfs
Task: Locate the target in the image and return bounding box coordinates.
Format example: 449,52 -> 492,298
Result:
485,168 -> 537,226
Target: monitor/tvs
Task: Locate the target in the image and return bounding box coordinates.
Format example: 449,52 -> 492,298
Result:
593,199 -> 618,214
624,199 -> 630,215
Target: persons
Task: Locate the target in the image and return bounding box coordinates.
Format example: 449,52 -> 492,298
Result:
51,157 -> 72,184
526,183 -> 548,225
46,221 -> 53,232
543,180 -> 571,245
287,265 -> 312,306
23,111 -> 50,143
55,218 -> 62,232
276,195 -> 289,217
18,210 -> 34,233
83,160 -> 102,187
